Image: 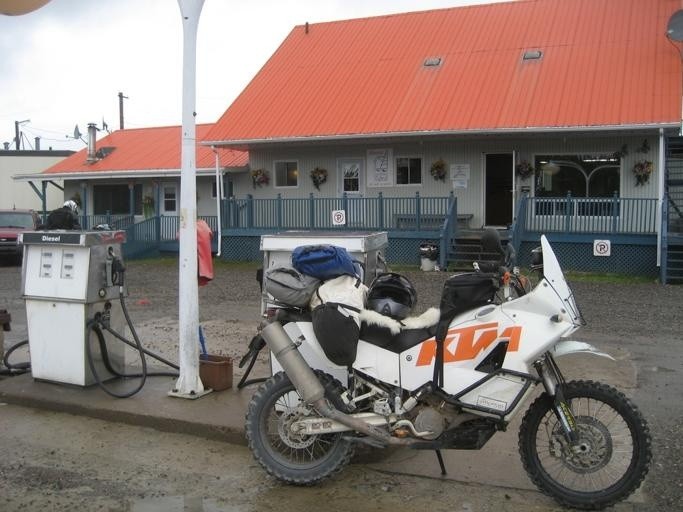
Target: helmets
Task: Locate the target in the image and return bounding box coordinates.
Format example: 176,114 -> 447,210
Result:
62,199 -> 79,214
367,271 -> 418,320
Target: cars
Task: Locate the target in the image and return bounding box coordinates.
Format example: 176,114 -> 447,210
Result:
1,209 -> 43,265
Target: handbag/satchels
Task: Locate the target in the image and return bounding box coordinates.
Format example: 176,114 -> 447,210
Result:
264,243 -> 371,366
441,272 -> 497,320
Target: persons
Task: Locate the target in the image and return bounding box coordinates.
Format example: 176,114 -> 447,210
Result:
43,199 -> 82,231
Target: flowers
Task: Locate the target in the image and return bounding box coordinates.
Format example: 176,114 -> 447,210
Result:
250,168 -> 268,186
633,159 -> 653,185
311,167 -> 328,189
517,158 -> 534,179
429,158 -> 449,181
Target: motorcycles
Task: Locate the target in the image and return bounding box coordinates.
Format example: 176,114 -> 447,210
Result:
245,234 -> 653,505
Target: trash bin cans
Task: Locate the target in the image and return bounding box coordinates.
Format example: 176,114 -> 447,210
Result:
420,243 -> 438,271
